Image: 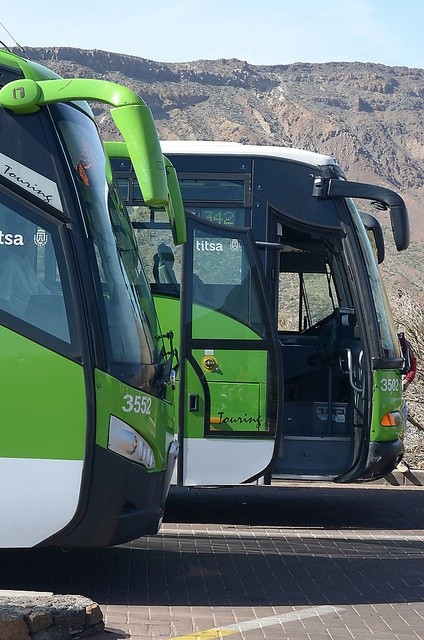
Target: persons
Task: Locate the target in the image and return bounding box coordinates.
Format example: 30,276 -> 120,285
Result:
0,233 -> 52,306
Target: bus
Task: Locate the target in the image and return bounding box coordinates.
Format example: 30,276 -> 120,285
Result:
0,50 -> 189,558
103,139 -> 412,490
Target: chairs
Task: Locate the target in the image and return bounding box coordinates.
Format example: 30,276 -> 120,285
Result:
154,244 -> 177,284
